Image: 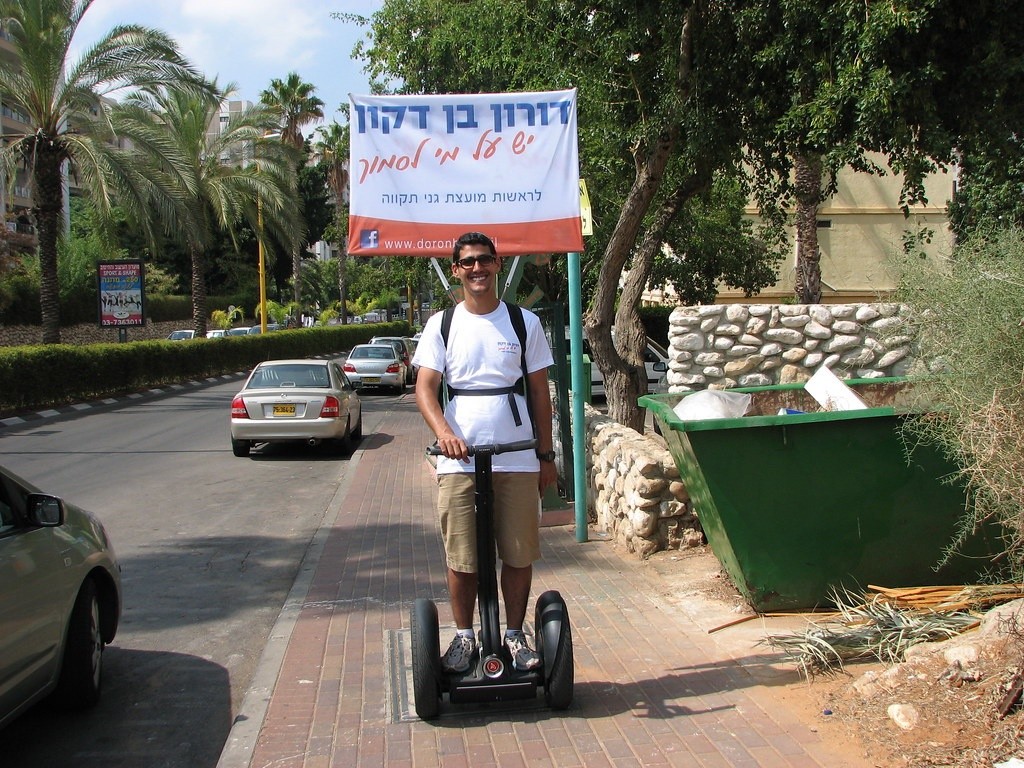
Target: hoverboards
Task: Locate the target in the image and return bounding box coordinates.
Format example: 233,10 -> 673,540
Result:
409,438 -> 574,722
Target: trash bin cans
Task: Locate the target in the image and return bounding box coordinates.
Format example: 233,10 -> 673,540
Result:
637,372 -> 1024,613
550,354 -> 592,404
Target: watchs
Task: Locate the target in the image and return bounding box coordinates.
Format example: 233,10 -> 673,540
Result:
537,451 -> 555,462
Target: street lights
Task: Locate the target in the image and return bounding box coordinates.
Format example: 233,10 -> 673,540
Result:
257,128 -> 280,335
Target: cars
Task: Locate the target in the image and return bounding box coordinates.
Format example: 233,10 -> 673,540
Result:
343,344 -> 409,395
229,326 -> 252,335
230,358 -> 364,457
348,316 -> 362,324
368,330 -> 423,383
1,464 -> 125,729
545,326 -> 669,398
165,329 -> 196,340
251,324 -> 286,334
207,330 -> 232,339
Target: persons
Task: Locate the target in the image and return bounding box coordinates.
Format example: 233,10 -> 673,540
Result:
228,303 -> 237,328
307,241 -> 315,257
302,306 -> 315,328
411,232 -> 557,674
101,291 -> 142,311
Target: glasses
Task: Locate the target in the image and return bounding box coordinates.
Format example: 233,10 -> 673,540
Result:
457,254 -> 497,270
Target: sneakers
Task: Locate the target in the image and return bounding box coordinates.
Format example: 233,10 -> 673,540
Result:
502,630 -> 541,670
442,630 -> 476,673
